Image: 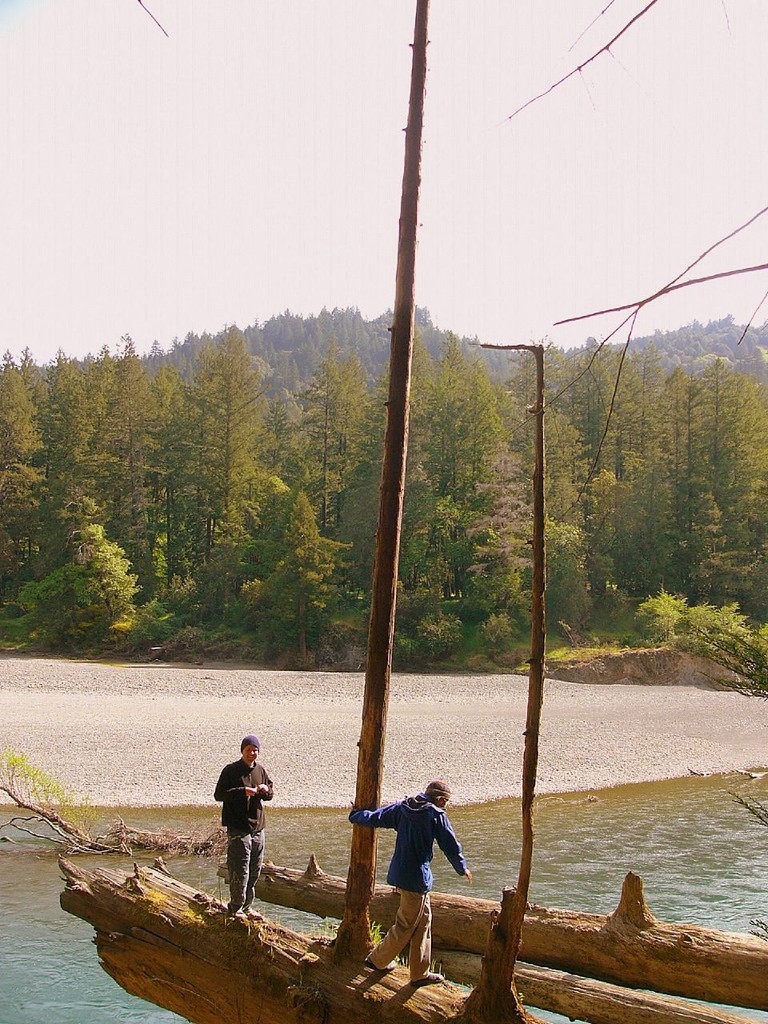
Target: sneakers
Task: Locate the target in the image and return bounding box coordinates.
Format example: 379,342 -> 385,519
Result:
412,972 -> 444,985
244,908 -> 262,919
229,911 -> 248,918
364,957 -> 397,972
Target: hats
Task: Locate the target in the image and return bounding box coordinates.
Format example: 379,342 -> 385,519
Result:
241,735 -> 260,754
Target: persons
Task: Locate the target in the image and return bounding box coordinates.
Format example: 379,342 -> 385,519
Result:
347,781 -> 473,987
213,735 -> 274,919
